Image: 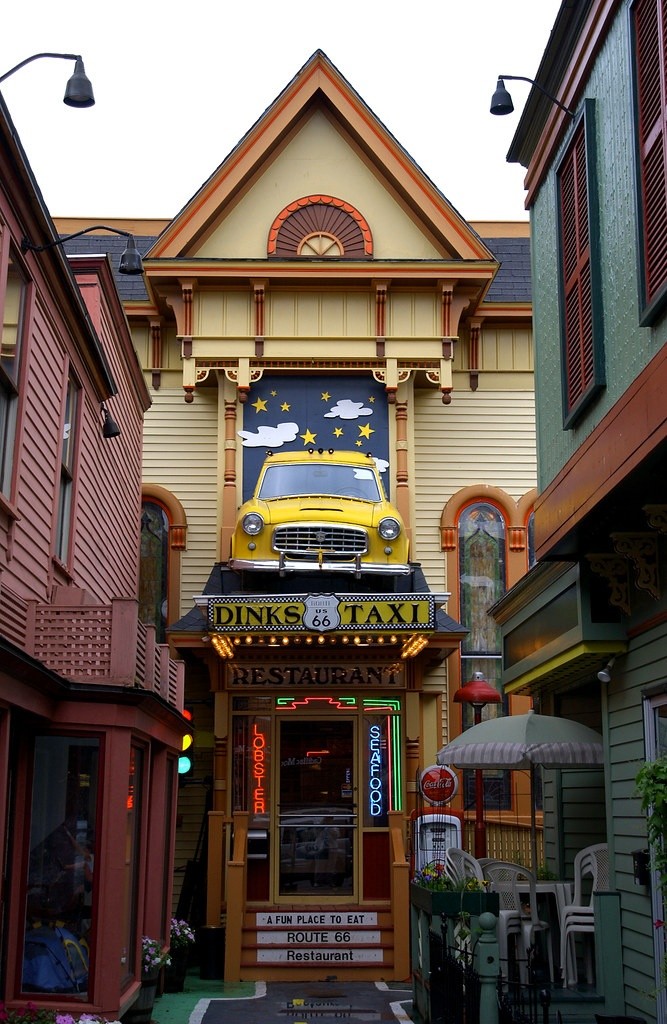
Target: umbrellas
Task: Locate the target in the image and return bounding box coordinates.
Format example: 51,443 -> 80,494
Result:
436,708 -> 603,883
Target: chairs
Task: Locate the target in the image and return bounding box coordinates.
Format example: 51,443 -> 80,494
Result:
445,842 -> 608,994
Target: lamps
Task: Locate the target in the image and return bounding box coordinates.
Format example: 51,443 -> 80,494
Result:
489,75 -> 577,123
100,403 -> 121,439
0,52 -> 96,108
21,226 -> 144,276
598,652 -> 620,682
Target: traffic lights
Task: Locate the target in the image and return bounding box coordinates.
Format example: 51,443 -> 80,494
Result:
178,709 -> 194,780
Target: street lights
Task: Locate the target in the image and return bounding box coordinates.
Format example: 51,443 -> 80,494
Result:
454,672 -> 504,860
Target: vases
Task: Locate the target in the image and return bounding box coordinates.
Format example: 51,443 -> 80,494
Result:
122,966 -> 160,1024
163,968 -> 188,994
409,879 -> 500,918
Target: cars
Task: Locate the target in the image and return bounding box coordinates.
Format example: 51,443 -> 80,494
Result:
230,447 -> 413,579
280,817 -> 351,858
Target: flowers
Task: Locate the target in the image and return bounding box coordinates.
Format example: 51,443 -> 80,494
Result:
0,999 -> 122,1024
142,935 -> 173,972
411,861 -> 489,892
169,918 -> 197,968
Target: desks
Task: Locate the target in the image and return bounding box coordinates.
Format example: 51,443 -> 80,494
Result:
487,880 -> 587,985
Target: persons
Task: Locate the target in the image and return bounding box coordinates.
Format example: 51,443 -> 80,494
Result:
56,824 -> 95,904
309,817 -> 341,892
